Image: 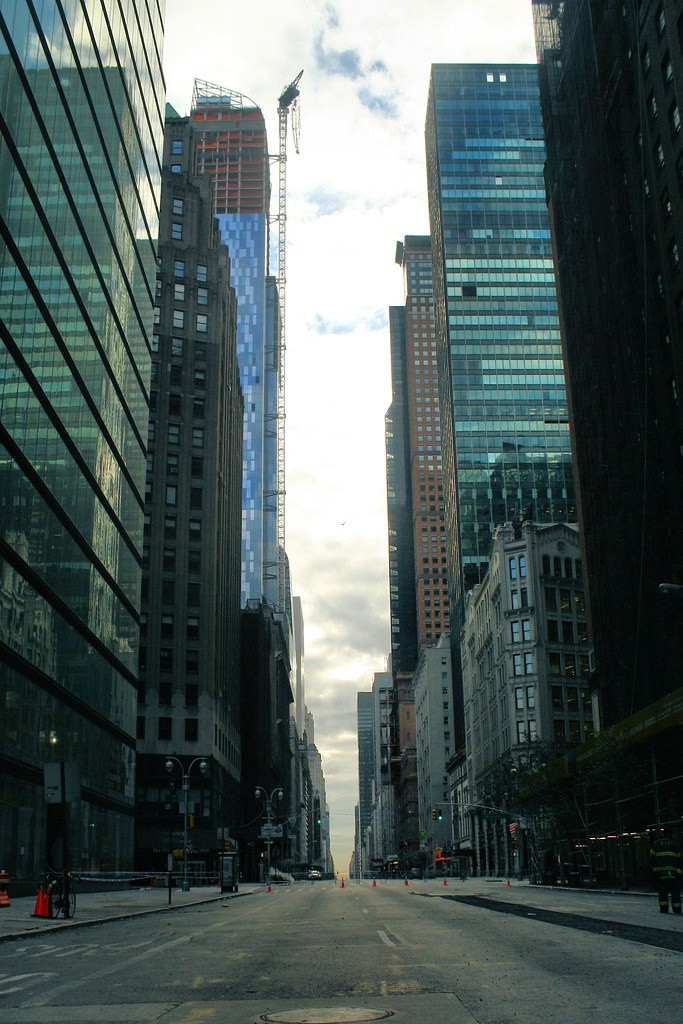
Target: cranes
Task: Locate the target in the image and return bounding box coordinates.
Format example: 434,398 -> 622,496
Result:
276,68 -> 303,614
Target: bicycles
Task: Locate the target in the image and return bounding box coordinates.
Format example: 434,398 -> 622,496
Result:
44,872 -> 76,919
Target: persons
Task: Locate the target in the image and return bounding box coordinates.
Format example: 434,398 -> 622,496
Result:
651,827 -> 683,914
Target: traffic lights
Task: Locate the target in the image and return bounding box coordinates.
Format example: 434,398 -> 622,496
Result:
438,810 -> 442,820
432,808 -> 437,820
317,814 -> 321,823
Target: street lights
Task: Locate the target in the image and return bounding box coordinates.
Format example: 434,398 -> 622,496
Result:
50,737 -> 71,919
166,756 -> 208,892
255,786 -> 283,886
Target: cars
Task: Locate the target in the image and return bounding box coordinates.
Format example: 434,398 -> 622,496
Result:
308,871 -> 321,879
409,868 -> 422,880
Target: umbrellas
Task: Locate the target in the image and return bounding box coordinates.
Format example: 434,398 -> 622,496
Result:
435,856 -> 453,880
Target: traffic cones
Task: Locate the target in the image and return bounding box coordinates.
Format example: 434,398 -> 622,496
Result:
42,887 -> 53,919
404,879 -> 409,886
340,880 -> 345,888
443,880 -> 447,886
266,880 -> 272,893
506,879 -> 511,887
30,883 -> 45,918
372,879 -> 377,887
